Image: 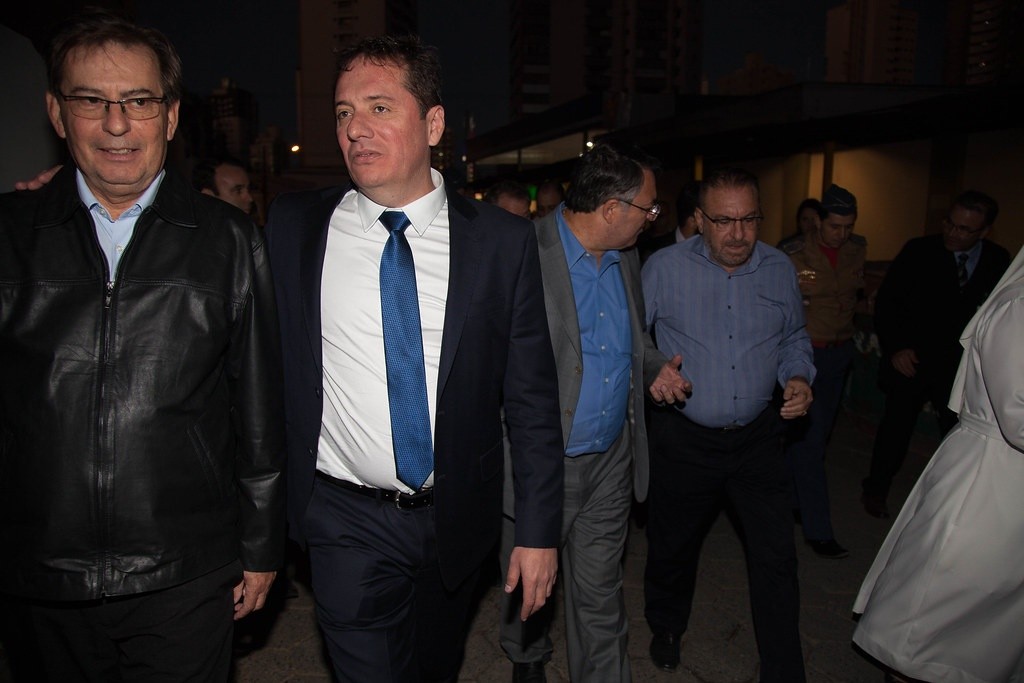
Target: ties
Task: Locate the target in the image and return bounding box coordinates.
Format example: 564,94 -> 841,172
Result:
376,209 -> 435,492
956,253 -> 970,290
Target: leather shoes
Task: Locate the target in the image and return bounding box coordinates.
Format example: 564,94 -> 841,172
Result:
650,633 -> 678,672
511,662 -> 546,683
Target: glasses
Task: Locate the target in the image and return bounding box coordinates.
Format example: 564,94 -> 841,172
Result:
56,85 -> 165,121
940,218 -> 982,235
602,197 -> 661,223
698,207 -> 764,229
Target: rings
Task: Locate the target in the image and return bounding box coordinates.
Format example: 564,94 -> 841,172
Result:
803,411 -> 807,417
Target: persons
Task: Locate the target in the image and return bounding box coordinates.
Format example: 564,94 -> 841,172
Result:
458,167 -> 574,221
853,245 -> 1024,683
0,20 -> 282,683
16,40 -> 566,683
193,152 -> 300,600
773,184 -> 865,556
641,168 -> 818,683
861,190 -> 1013,519
499,144 -> 692,683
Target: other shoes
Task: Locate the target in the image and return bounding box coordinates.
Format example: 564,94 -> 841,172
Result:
859,493 -> 890,519
812,541 -> 848,560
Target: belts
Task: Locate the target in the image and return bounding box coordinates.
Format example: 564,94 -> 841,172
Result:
315,471 -> 446,512
